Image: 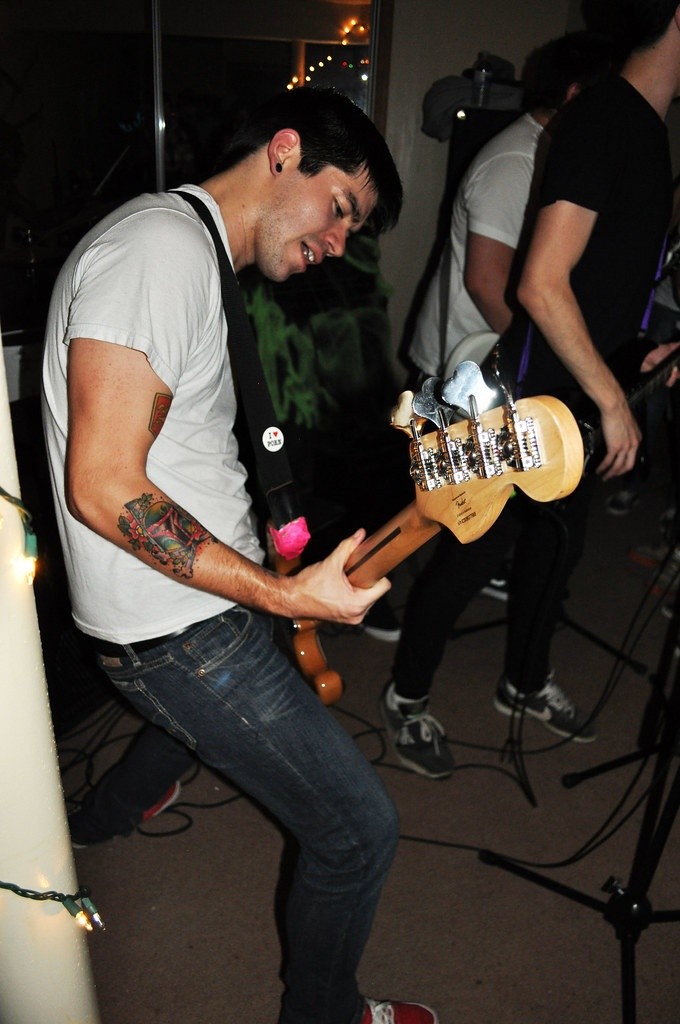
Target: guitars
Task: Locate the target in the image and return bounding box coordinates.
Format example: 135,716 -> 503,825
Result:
523,346 -> 680,538
263,340 -> 585,705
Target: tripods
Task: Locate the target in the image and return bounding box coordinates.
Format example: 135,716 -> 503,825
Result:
478,639 -> 680,1024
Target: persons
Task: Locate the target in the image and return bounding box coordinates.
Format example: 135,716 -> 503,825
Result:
606,242 -> 680,659
408,32 -> 606,603
36,90 -> 439,1024
377,0 -> 680,778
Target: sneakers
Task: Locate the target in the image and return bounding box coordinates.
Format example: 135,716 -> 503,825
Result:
363,996 -> 439,1024
379,683 -> 451,778
71,777 -> 182,850
492,673 -> 599,743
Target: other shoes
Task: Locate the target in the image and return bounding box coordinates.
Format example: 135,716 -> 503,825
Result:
605,488 -> 641,518
359,592 -> 402,643
629,542 -> 670,565
647,548 -> 680,593
